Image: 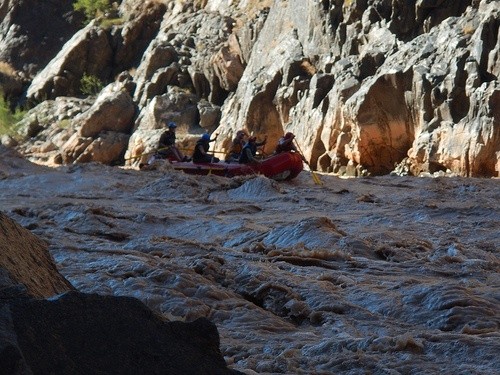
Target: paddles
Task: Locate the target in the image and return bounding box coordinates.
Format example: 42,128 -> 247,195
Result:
207,133 -> 219,177
123,145 -> 181,160
180,147 -> 225,154
257,135 -> 268,178
293,136 -> 320,185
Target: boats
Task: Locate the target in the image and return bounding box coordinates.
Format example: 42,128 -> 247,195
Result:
137,149 -> 304,181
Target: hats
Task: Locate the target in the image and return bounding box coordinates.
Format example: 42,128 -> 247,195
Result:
167,122 -> 178,128
248,135 -> 256,140
202,133 -> 210,141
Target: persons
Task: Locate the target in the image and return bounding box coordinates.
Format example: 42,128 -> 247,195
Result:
238,134 -> 269,164
273,132 -> 309,163
228,131 -> 249,162
193,133 -> 220,163
158,122 -> 191,162
